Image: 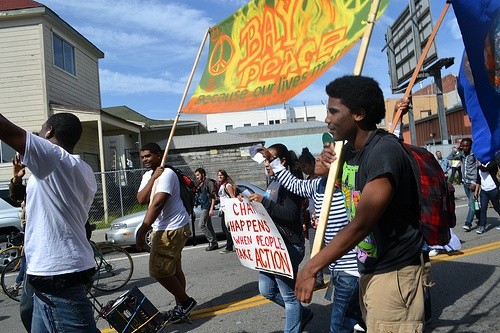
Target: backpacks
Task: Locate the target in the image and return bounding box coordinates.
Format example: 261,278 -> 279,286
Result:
204,178 -> 220,205
351,132 -> 456,245
165,164 -> 195,215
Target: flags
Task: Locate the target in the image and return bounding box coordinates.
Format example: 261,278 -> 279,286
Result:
446,0 -> 500,166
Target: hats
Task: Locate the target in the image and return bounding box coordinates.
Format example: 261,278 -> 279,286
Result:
322,132 -> 348,147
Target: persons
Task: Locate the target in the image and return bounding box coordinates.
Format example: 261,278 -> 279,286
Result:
436,137 -> 500,235
136,142 -> 197,325
237,94 -> 412,333
294,76 -> 435,333
194,167 -> 236,254
0,112 -> 102,333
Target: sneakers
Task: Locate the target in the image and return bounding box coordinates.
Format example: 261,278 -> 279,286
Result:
476,226 -> 486,233
220,249 -> 233,254
169,296 -> 197,323
205,242 -> 218,250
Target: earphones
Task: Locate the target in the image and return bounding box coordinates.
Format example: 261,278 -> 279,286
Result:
45,131 -> 50,136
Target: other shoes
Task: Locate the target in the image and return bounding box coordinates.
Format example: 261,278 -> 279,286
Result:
463,225 -> 471,231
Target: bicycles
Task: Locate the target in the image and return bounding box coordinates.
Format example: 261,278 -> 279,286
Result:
1,240 -> 134,303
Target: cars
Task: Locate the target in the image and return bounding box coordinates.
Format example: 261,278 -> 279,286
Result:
102,181 -> 269,254
0,180 -> 94,274
